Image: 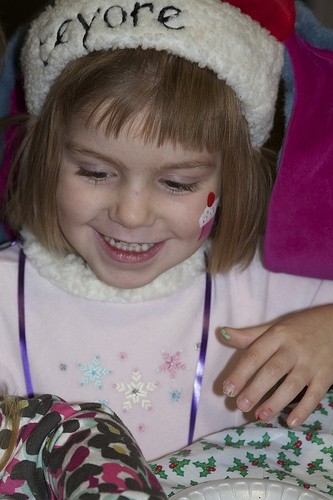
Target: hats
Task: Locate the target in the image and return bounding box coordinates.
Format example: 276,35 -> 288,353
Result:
20,0 -> 286,150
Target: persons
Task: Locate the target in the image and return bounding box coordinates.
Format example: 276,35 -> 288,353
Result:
0,393 -> 168,500
0,1 -> 332,462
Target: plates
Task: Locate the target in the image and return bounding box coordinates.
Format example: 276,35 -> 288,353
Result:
168,478 -> 331,500
